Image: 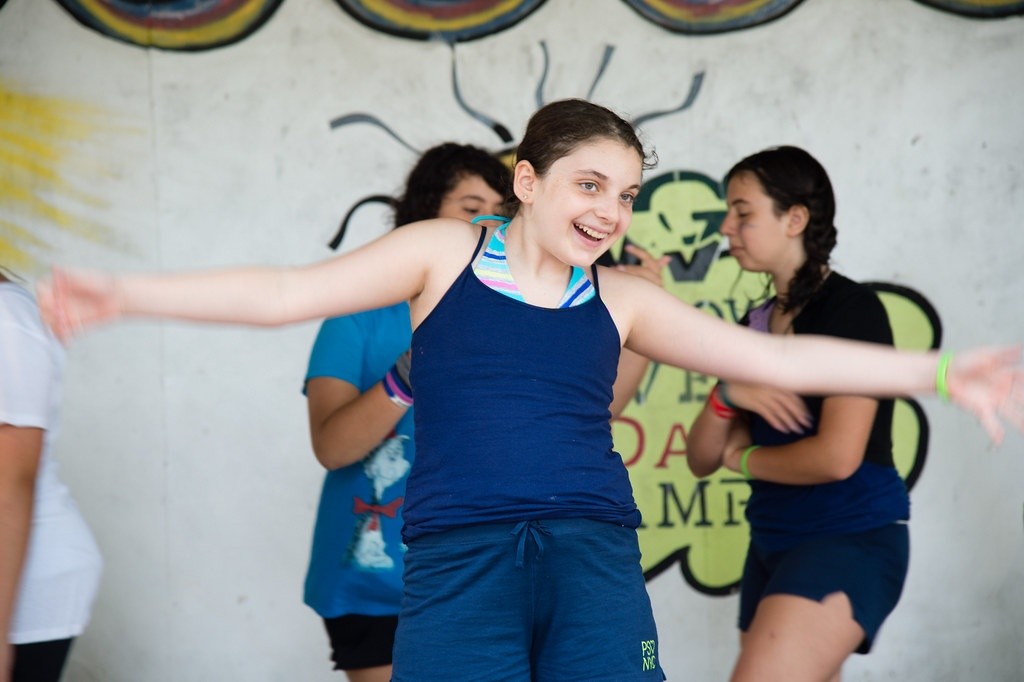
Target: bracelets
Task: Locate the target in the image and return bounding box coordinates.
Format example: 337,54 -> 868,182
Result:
382,348 -> 417,409
934,347 -> 955,405
706,382 -> 740,420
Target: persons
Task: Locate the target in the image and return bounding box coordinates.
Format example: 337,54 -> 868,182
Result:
37,98 -> 1022,682
682,140 -> 910,681
298,143 -> 672,682
1,267 -> 103,682
